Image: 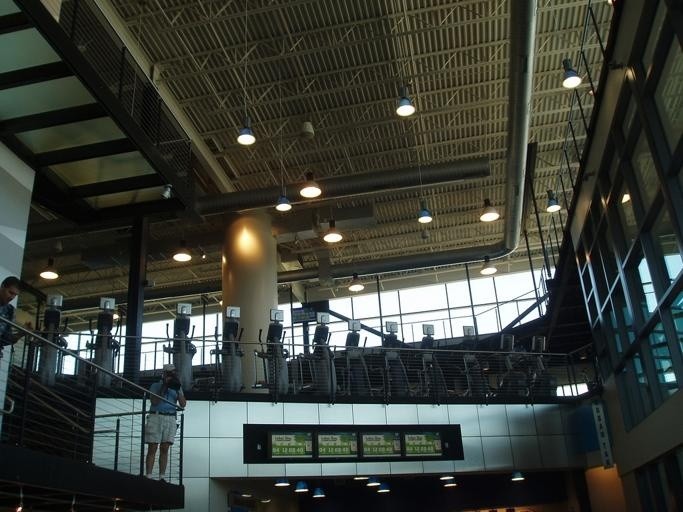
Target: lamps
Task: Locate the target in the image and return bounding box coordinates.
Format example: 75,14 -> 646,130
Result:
297,144 -> 326,200
237,1 -> 261,147
544,187 -> 563,215
39,259 -> 59,280
415,163 -> 437,225
347,269 -> 367,293
560,46 -> 583,91
477,178 -> 501,224
478,250 -> 501,277
172,220 -> 192,262
322,215 -> 345,245
275,60 -> 294,214
228,471 -> 525,503
395,94 -> 419,119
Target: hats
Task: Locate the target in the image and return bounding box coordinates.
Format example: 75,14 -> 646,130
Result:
161,363 -> 178,373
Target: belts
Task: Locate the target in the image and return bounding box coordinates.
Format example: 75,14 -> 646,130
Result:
148,410 -> 177,417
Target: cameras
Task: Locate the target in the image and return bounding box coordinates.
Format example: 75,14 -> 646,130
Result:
165,373 -> 181,390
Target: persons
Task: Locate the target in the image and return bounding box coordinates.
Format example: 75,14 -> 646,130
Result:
143,364 -> 185,483
0,276 -> 33,358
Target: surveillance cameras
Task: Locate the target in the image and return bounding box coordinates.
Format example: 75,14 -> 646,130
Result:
299,121 -> 315,139
420,229 -> 430,240
160,187 -> 171,200
